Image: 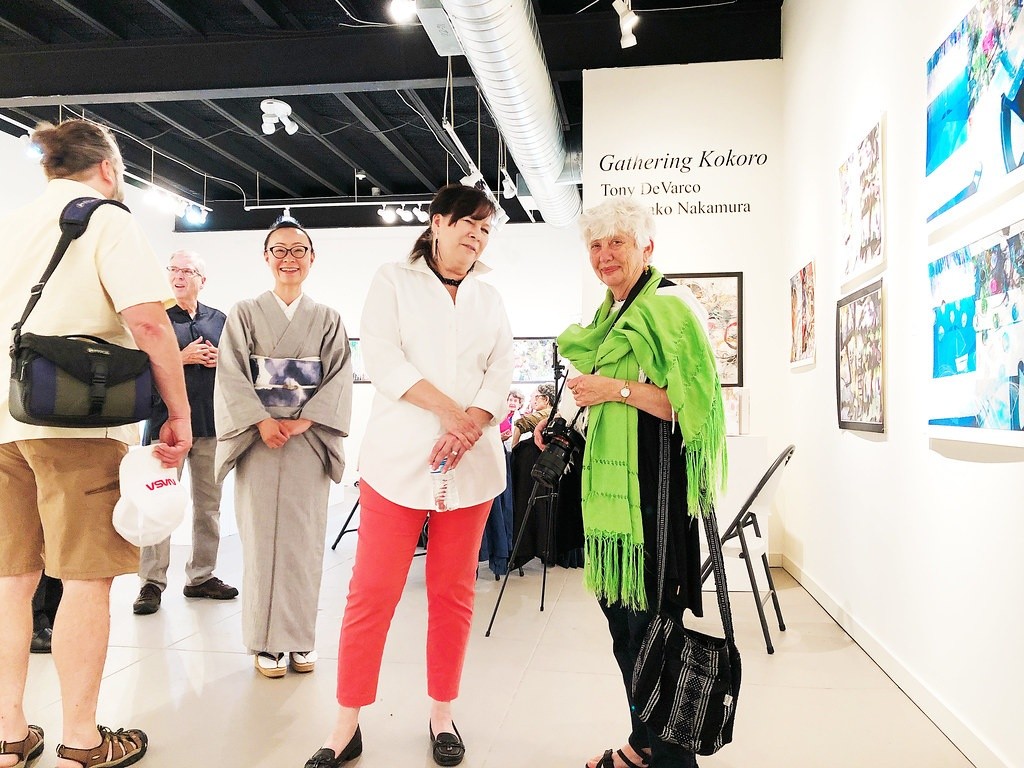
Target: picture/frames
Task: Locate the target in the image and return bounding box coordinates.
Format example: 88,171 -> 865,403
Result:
787,259 -> 816,368
664,271 -> 743,387
511,337 -> 558,384
833,111 -> 887,294
836,277 -> 885,434
348,338 -> 371,383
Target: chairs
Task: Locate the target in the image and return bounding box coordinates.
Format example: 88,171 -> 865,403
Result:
702,443 -> 797,655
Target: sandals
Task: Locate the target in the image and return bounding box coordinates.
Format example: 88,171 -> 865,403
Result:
0,724 -> 44,768
56,725 -> 148,768
585,746 -> 651,768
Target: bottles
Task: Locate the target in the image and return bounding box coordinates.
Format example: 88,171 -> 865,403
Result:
430,438 -> 460,513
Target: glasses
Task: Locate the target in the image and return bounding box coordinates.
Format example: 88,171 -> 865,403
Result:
267,246 -> 308,258
167,266 -> 202,277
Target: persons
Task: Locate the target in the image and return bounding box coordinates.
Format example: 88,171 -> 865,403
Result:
214,221 -> 354,678
131,244 -> 238,616
0,118 -> 193,768
534,198 -> 728,768
303,184 -> 516,768
499,383 -> 563,452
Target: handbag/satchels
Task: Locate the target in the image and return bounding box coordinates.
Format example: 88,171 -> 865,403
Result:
6,333 -> 155,429
631,613 -> 742,756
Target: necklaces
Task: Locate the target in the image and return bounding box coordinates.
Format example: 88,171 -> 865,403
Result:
424,255 -> 463,287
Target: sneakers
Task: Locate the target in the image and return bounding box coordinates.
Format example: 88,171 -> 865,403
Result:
184,576 -> 238,599
133,584 -> 162,614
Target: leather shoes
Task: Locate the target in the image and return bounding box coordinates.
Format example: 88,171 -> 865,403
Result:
30,628 -> 53,652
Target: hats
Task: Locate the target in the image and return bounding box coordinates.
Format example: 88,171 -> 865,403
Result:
112,443 -> 185,547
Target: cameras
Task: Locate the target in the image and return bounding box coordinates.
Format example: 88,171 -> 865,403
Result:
531,417 -> 586,489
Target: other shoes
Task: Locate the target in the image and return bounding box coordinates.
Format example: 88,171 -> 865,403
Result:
305,725 -> 362,768
429,718 -> 465,765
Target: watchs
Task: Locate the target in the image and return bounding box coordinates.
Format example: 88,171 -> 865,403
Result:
620,379 -> 631,403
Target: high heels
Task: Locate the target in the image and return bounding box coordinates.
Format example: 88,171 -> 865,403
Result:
253,651 -> 286,677
289,649 -> 314,672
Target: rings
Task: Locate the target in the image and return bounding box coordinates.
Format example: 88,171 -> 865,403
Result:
571,387 -> 577,395
451,451 -> 458,456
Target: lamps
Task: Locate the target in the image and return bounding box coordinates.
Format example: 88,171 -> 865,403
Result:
502,178 -> 515,199
620,22 -> 637,49
377,204 -> 396,224
396,204 -> 413,222
489,203 -> 510,231
612,0 -> 639,29
459,163 -> 483,188
412,204 -> 429,223
356,169 -> 367,180
260,99 -> 298,135
